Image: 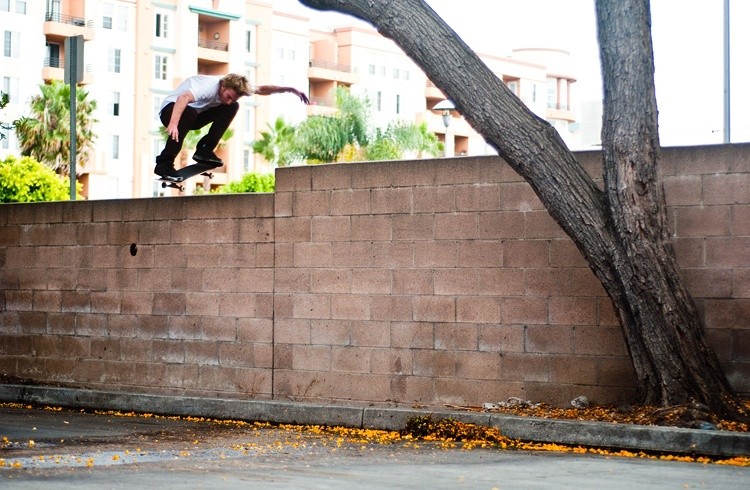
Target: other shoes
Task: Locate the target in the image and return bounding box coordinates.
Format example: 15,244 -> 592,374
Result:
192,150 -> 223,165
154,164 -> 183,182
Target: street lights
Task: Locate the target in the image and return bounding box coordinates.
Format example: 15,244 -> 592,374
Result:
432,99 -> 456,156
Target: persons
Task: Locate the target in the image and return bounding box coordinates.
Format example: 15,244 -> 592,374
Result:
152,73 -> 310,181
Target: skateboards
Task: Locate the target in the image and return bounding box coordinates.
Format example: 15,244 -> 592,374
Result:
155,158 -> 224,192
